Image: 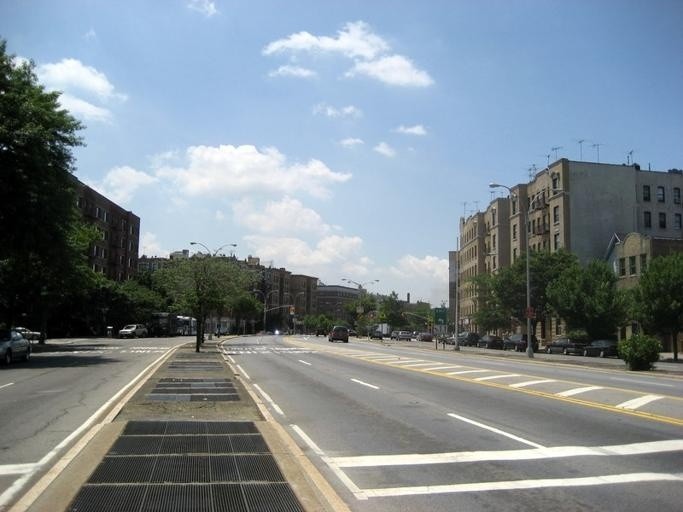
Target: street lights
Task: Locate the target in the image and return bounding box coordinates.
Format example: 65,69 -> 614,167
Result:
283,291 -> 305,332
189,241 -> 238,340
250,289 -> 280,333
486,180 -> 572,357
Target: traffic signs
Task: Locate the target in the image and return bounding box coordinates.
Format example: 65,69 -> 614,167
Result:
428,303 -> 452,332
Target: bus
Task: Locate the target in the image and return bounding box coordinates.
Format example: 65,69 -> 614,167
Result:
151,309 -> 197,337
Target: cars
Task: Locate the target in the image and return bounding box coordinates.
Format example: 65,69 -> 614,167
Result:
327,325 -> 349,344
118,324 -> 149,337
315,326 -> 332,337
0,325 -> 47,368
370,329 -> 619,359
341,276 -> 379,334
